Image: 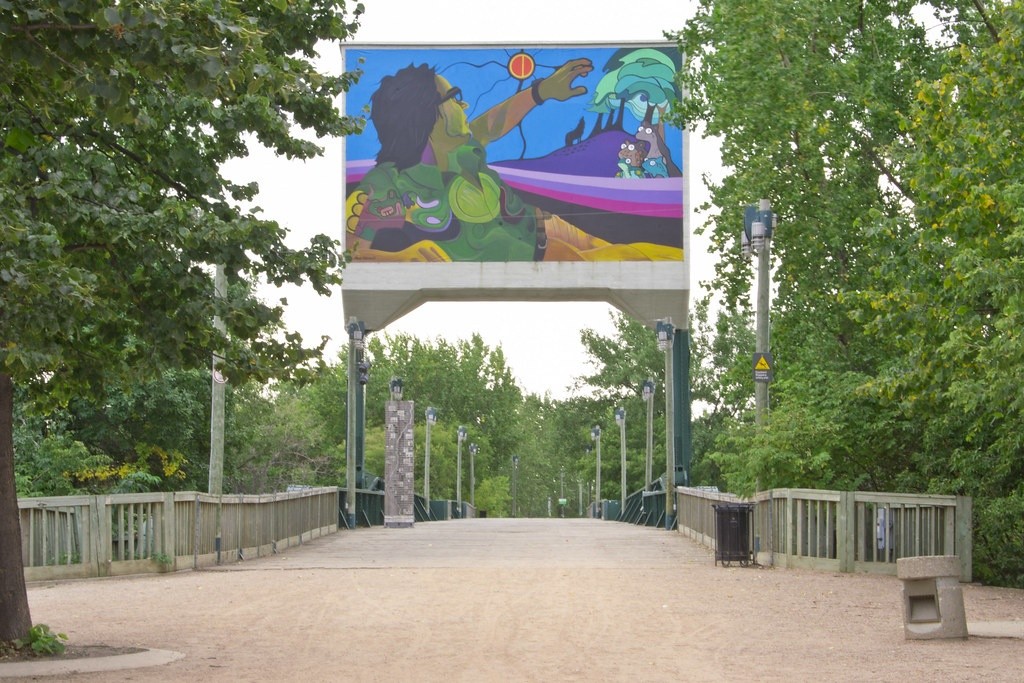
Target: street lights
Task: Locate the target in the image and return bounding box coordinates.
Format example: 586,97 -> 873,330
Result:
642,376 -> 654,492
455,424 -> 467,519
344,314 -> 371,531
424,406 -> 437,518
590,424 -> 603,518
468,442 -> 481,506
512,454 -> 519,517
740,197 -> 777,493
655,315 -> 679,530
614,405 -> 627,514
560,465 -> 566,499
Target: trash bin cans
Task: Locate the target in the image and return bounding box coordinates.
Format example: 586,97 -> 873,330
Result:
608,500 -> 619,520
710,502 -> 758,569
